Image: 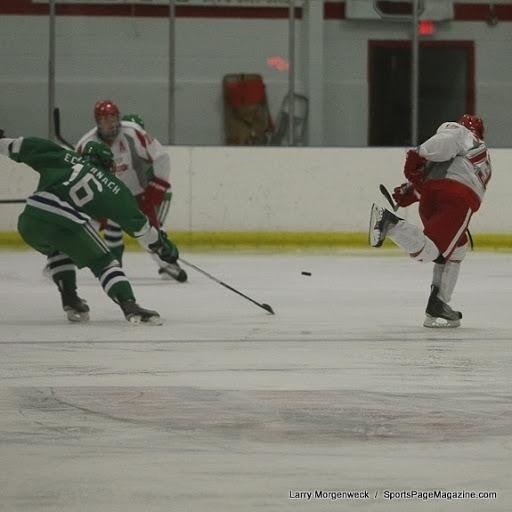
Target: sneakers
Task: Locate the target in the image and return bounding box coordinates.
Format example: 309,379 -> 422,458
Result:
159,263 -> 187,282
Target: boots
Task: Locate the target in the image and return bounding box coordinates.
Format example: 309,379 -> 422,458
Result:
426,284 -> 462,321
111,294 -> 159,321
56,278 -> 90,312
375,208 -> 405,247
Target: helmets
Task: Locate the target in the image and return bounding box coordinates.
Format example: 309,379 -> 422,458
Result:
460,114 -> 485,140
83,140 -> 117,175
123,114 -> 144,126
95,100 -> 121,137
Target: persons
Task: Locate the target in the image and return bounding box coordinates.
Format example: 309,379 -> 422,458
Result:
368,113 -> 492,322
8,99 -> 187,326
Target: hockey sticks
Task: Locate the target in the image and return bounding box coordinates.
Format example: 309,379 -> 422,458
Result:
177,257 -> 273,314
379,185 -> 415,213
53,107 -> 77,153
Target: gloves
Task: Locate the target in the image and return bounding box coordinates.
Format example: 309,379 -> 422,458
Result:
150,231 -> 178,263
144,177 -> 169,205
402,149 -> 423,193
392,182 -> 421,207
